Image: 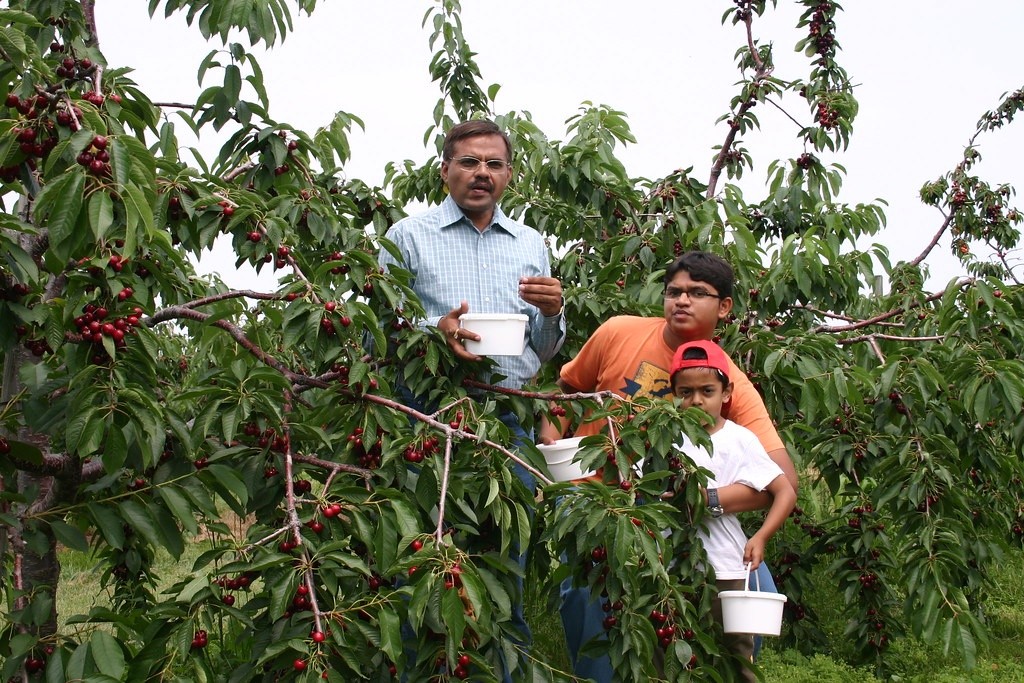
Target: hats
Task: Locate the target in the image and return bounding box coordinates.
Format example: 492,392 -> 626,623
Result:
670,340 -> 732,418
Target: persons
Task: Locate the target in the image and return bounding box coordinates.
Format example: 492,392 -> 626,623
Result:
362,119 -> 566,683
537,251 -> 799,682
632,338 -> 797,682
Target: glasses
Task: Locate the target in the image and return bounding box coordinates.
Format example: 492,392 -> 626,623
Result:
660,286 -> 723,301
447,156 -> 510,174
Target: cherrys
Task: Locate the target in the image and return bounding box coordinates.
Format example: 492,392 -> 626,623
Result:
6,2 -> 1017,683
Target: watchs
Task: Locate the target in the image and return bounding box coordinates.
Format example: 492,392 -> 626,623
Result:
707,488 -> 723,519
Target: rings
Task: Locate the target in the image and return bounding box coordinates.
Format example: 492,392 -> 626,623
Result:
454,329 -> 459,339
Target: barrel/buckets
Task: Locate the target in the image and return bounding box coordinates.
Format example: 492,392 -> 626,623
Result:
718,561 -> 788,636
459,314 -> 527,356
534,435 -> 597,482
